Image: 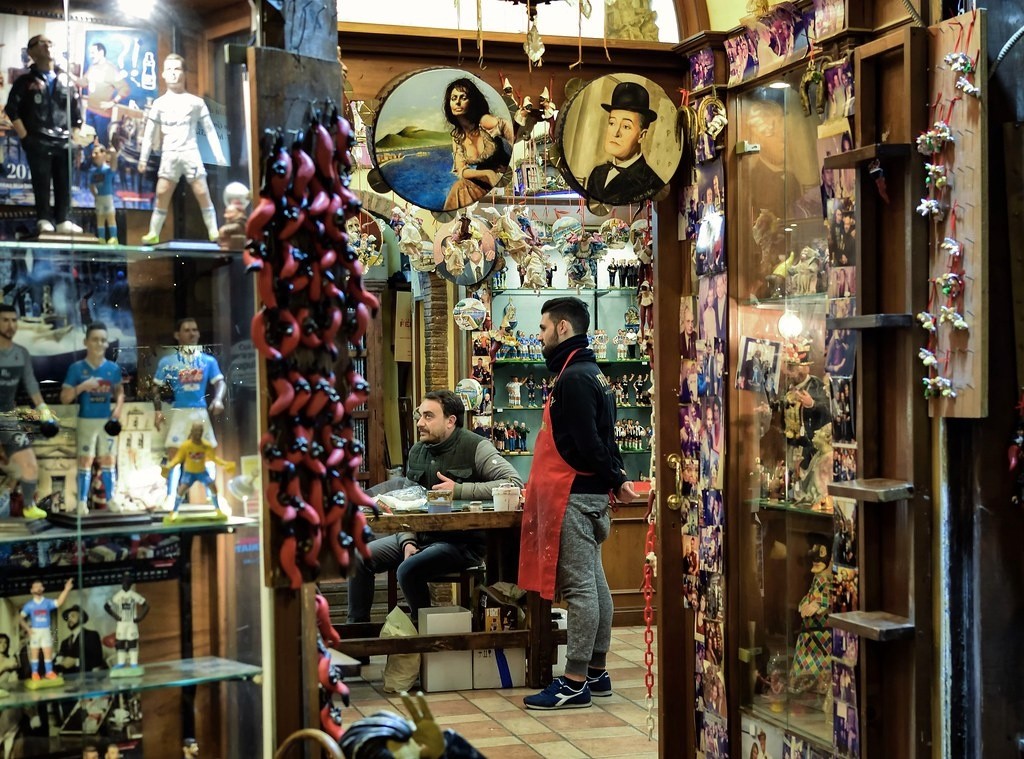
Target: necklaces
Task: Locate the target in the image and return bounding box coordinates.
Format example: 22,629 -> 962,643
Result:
64,42 -> 128,169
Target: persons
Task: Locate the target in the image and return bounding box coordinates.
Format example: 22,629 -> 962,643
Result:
678,103 -> 726,759
690,0 -> 841,92
750,336 -> 832,506
56,605 -> 109,673
346,217 -> 374,270
822,67 -> 857,758
750,729 -> 804,759
348,206 -> 424,274
0,633 -> 21,688
436,235 -> 495,440
747,99 -> 800,265
489,207 -> 652,483
0,0 -> 248,759
519,297 -> 639,710
442,78 -> 514,210
587,81 -> 666,204
341,390 -> 523,664
17,629 -> 54,679
747,350 -> 770,387
339,691 -> 488,759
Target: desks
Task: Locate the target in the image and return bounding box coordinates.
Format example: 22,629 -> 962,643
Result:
331,505 -> 568,689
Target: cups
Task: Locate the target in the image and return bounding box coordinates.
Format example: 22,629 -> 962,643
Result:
470,501 -> 483,512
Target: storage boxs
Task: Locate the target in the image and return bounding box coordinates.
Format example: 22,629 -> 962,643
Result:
473,648 -> 526,689
418,606 -> 473,693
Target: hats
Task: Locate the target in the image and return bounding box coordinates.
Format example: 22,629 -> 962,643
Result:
601,82 -> 658,123
60,603 -> 87,621
473,582 -> 528,624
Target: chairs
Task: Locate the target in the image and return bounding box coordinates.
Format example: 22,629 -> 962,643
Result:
388,562 -> 487,613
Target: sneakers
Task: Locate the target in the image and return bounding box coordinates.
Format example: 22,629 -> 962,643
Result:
523,677 -> 593,710
586,671 -> 613,696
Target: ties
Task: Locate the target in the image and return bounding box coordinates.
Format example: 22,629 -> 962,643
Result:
68,634 -> 73,648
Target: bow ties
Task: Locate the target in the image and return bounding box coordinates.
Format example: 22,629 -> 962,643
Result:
606,160 -> 627,175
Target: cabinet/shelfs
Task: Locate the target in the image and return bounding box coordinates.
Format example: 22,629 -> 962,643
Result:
0,0 -> 275,759
735,50 -> 859,759
489,225 -> 651,456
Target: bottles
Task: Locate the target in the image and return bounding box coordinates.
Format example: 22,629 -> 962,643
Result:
491,482 -> 520,511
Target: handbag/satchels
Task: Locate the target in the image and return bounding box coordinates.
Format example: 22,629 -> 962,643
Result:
376,603 -> 422,695
